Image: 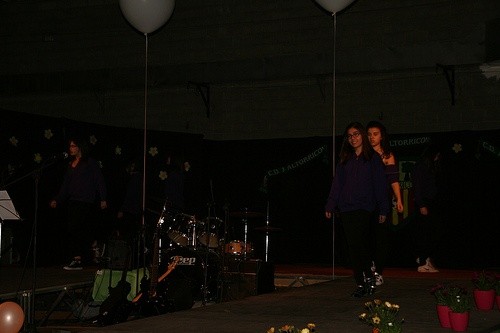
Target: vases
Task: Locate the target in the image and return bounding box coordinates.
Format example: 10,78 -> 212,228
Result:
473,288 -> 496,312
449,311 -> 470,332
436,304 -> 452,329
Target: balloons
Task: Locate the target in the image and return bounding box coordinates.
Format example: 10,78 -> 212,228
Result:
0,302 -> 25,333
315,0 -> 354,13
119,0 -> 175,34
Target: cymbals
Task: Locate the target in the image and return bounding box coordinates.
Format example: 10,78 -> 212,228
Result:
231,212 -> 265,218
147,207 -> 168,219
254,227 -> 280,231
150,196 -> 177,208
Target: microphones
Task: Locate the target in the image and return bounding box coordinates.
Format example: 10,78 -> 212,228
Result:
53,152 -> 69,159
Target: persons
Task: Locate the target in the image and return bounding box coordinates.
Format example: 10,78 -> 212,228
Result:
98,281 -> 136,326
409,147 -> 443,273
50,136 -> 107,270
326,122 -> 390,290
364,120 -> 403,285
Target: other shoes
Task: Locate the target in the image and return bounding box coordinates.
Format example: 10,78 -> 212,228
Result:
366,281 -> 375,296
352,285 -> 367,298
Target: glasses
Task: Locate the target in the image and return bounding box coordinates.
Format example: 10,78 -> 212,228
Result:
346,133 -> 361,139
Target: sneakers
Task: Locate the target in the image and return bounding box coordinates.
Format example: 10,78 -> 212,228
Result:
418,265 -> 439,273
93,241 -> 105,262
376,275 -> 383,285
416,257 -> 436,269
63,260 -> 83,270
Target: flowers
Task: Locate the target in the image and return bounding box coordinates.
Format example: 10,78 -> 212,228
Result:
359,299 -> 406,333
431,280 -> 473,312
264,320 -> 320,333
476,272 -> 500,295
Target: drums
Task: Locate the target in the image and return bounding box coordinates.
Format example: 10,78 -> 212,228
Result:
167,213 -> 197,246
163,248 -> 204,271
224,240 -> 255,261
198,217 -> 225,248
